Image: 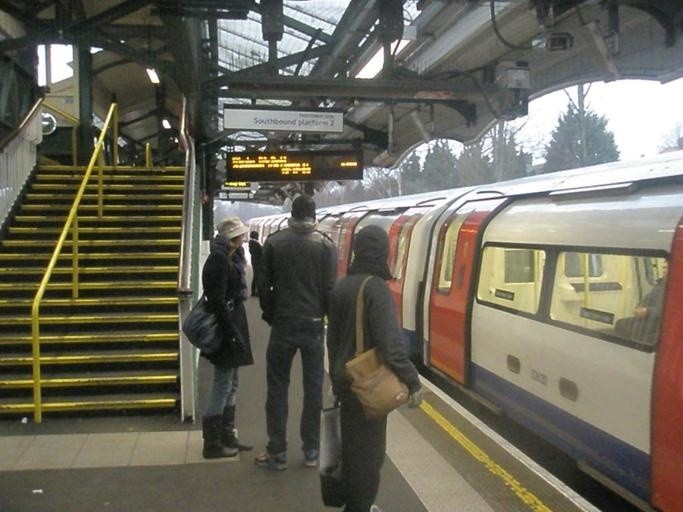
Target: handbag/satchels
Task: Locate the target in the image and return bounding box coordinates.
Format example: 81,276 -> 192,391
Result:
181,294 -> 235,354
313,403 -> 350,508
343,342 -> 413,420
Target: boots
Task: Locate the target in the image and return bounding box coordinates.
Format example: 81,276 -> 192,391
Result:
250,282 -> 256,296
200,412 -> 240,459
220,404 -> 255,451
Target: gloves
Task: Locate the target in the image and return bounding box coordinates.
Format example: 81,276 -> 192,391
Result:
407,387 -> 425,410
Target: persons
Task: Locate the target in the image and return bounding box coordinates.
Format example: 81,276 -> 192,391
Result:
254,194 -> 337,470
249,230 -> 263,296
202,217 -> 254,457
320,225 -> 424,510
614,259 -> 668,342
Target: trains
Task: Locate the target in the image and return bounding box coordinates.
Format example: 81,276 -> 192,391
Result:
243,146 -> 683,511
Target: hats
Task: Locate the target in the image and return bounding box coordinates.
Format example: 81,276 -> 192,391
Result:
351,223 -> 391,267
215,214 -> 251,241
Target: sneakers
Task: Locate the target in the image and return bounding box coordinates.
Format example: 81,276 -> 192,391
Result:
253,449 -> 290,472
302,445 -> 322,467
